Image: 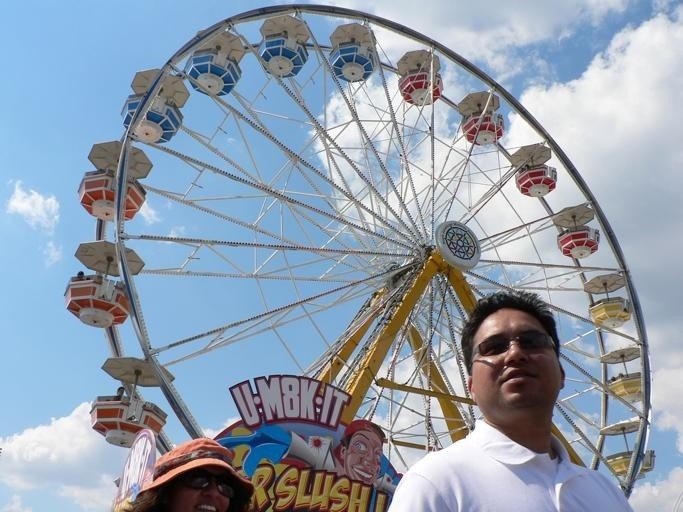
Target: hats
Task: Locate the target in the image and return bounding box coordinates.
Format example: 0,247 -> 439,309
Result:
136,437 -> 254,500
333,419 -> 384,467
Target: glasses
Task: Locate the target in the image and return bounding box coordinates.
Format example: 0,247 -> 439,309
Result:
182,469 -> 236,499
469,330 -> 558,357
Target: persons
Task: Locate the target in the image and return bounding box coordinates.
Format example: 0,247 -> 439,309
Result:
386,290 -> 635,512
333,419 -> 386,486
113,387 -> 125,400
123,437 -> 255,512
